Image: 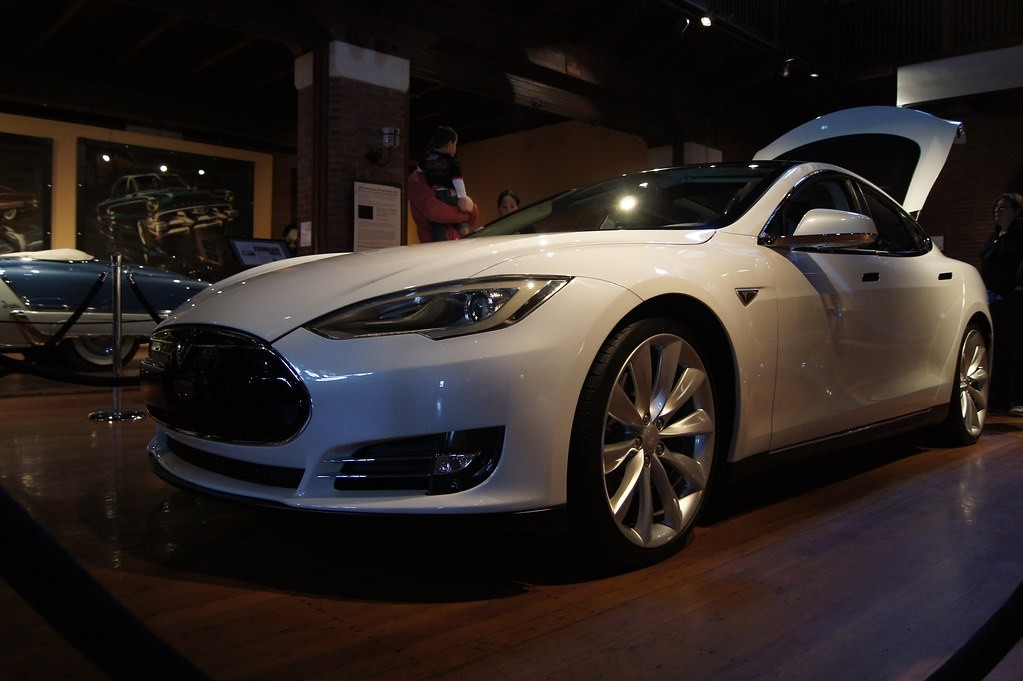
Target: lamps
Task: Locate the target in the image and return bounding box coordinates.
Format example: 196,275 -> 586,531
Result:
365,126 -> 404,169
778,53 -> 823,81
672,14 -> 715,35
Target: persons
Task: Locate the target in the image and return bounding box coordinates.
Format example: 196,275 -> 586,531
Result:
417,126 -> 469,242
408,140 -> 479,243
981,193 -> 1023,417
498,190 -> 535,235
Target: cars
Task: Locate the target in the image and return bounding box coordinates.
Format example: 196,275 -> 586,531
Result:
0,247 -> 212,369
139,105 -> 995,561
96,173 -> 242,247
